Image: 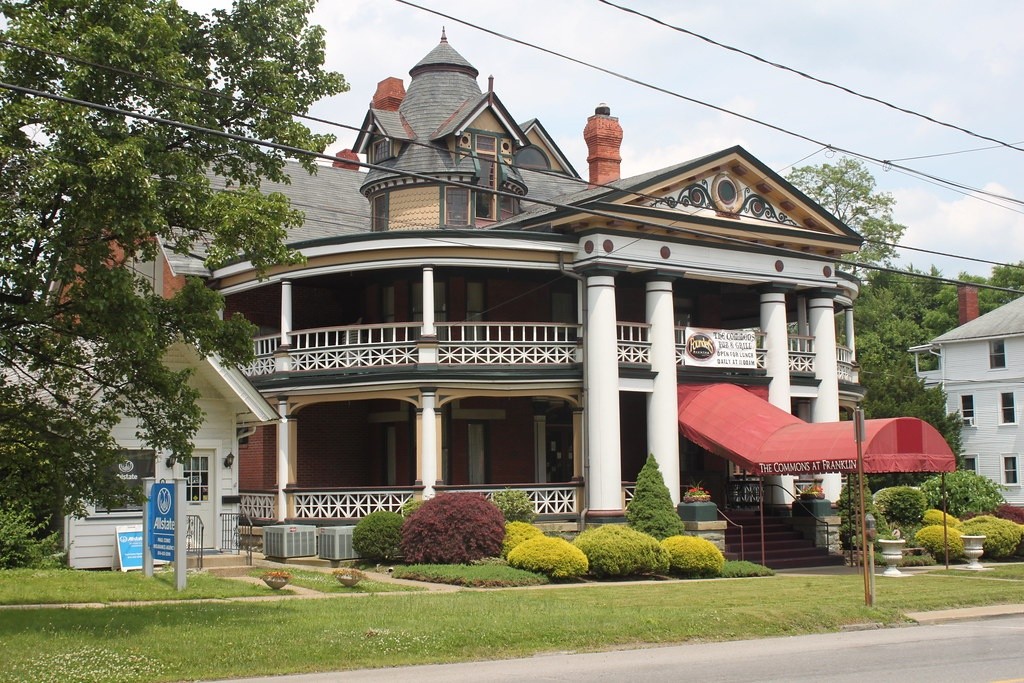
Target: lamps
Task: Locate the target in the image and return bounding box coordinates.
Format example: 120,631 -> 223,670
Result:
166,453 -> 177,468
224,452 -> 234,468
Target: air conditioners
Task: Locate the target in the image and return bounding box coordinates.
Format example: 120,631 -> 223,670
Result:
962,417 -> 972,426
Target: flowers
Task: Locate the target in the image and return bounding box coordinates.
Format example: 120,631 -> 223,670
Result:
685,480 -> 709,496
803,483 -> 823,498
259,570 -> 292,582
332,569 -> 370,581
878,520 -> 900,540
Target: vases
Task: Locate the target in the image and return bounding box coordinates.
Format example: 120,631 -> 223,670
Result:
683,495 -> 712,503
801,493 -> 825,500
263,576 -> 290,589
336,574 -> 362,587
878,538 -> 906,575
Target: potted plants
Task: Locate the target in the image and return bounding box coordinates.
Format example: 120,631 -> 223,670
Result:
960,523 -> 987,569
